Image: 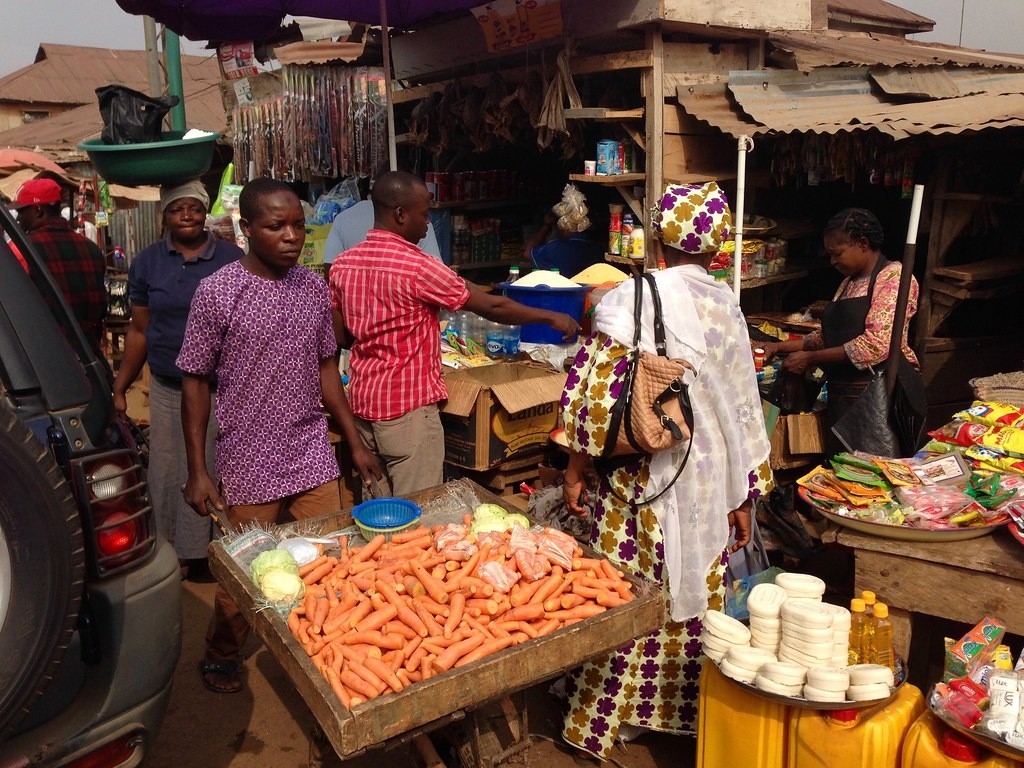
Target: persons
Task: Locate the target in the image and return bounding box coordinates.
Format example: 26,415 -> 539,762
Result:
547,182 -> 771,760
4,178 -> 107,357
523,200 -> 602,276
329,171 -> 583,505
111,180 -> 247,580
323,160 -> 443,280
755,209 -> 919,460
172,178 -> 381,694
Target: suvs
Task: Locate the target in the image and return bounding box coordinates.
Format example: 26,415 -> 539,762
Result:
1,204 -> 181,768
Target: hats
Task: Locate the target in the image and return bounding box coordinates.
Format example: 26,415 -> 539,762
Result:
5,178 -> 62,209
649,182 -> 732,253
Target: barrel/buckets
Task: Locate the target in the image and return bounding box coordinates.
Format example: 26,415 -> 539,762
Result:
588,292 -> 606,333
696,658 -> 791,768
770,367 -> 823,415
579,284 -> 599,336
788,681 -> 928,767
499,281 -> 590,346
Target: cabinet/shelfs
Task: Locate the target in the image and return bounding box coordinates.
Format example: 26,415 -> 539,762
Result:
907,156 -> 1024,435
663,170 -> 835,291
562,108 -> 646,265
395,133 -> 538,271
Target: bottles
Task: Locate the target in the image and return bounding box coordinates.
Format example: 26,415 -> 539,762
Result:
550,268 -> 560,274
458,309 -> 473,339
447,308 -> 459,344
847,598 -> 869,667
861,590 -> 876,664
532,269 -> 540,272
900,707 -> 1024,768
438,307 -> 448,343
865,603 -> 894,674
583,160 -> 644,259
472,312 -> 486,349
501,324 -> 521,362
503,266 -> 520,297
485,320 -> 503,359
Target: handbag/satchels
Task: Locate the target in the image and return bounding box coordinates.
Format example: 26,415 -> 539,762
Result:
95,85 -> 180,145
612,273 -> 696,455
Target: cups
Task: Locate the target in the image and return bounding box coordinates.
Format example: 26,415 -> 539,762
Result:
754,348 -> 764,372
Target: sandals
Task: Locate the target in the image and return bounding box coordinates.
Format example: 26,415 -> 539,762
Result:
201,648 -> 243,692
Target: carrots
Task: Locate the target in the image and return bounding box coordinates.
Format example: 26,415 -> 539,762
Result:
287,513 -> 635,710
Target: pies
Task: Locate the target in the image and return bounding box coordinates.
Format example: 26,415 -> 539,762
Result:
700,571 -> 895,702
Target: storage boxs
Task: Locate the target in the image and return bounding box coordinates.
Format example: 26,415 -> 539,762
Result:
439,361 -> 569,471
768,411 -> 828,470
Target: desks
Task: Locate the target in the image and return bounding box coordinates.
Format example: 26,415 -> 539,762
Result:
821,527 -> 1024,664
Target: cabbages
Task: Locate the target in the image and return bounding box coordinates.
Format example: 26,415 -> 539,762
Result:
249,548 -> 303,603
469,503 -> 529,535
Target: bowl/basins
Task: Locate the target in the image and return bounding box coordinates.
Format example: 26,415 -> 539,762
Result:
730,212 -> 778,236
76,129 -> 222,184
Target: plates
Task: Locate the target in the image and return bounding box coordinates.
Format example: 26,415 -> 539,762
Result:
1007,522 -> 1024,545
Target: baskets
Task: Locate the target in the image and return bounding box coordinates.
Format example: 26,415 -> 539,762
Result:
351,498 -> 422,541
